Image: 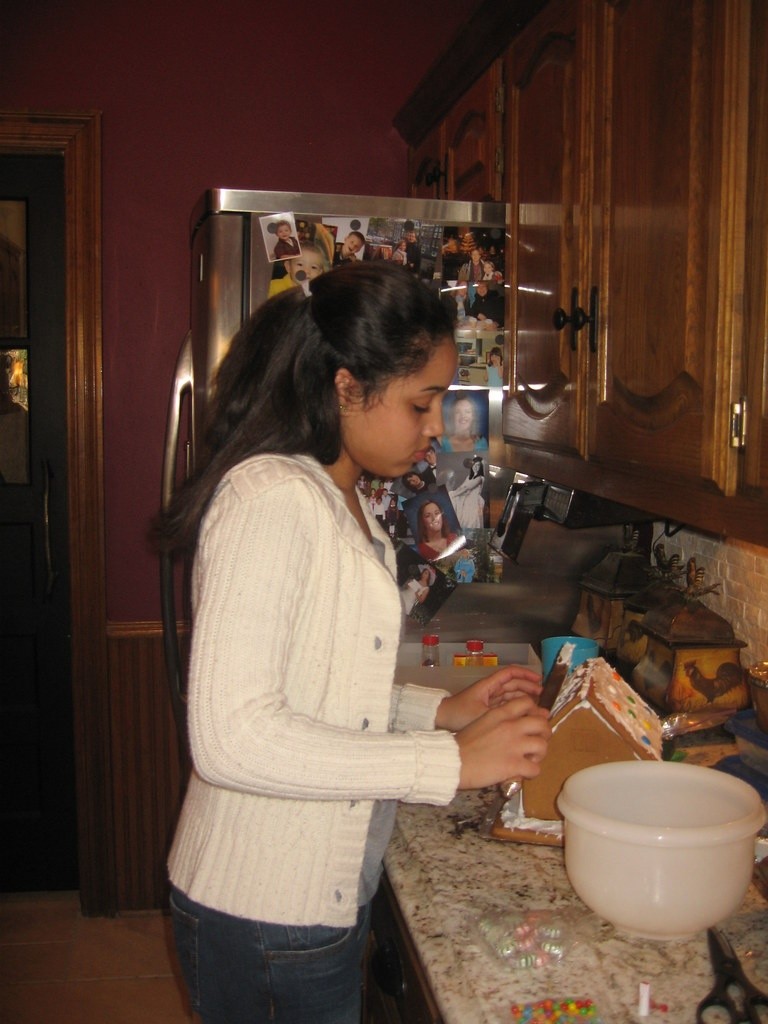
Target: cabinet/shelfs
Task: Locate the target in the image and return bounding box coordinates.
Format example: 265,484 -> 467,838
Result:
407,57 -> 500,202
502,0 -> 767,549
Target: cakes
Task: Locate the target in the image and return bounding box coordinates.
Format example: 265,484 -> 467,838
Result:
500,658 -> 666,829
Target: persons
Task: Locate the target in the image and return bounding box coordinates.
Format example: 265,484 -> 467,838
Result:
265,216 -> 505,615
0,354 -> 30,484
148,259 -> 550,1024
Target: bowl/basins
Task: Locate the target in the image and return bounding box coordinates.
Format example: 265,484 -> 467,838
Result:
556,760 -> 768,940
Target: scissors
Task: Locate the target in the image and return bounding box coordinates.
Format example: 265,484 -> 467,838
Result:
695,927 -> 768,1024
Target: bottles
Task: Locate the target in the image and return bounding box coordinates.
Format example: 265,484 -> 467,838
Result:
421,635 -> 440,666
455,314 -> 476,365
465,640 -> 484,666
